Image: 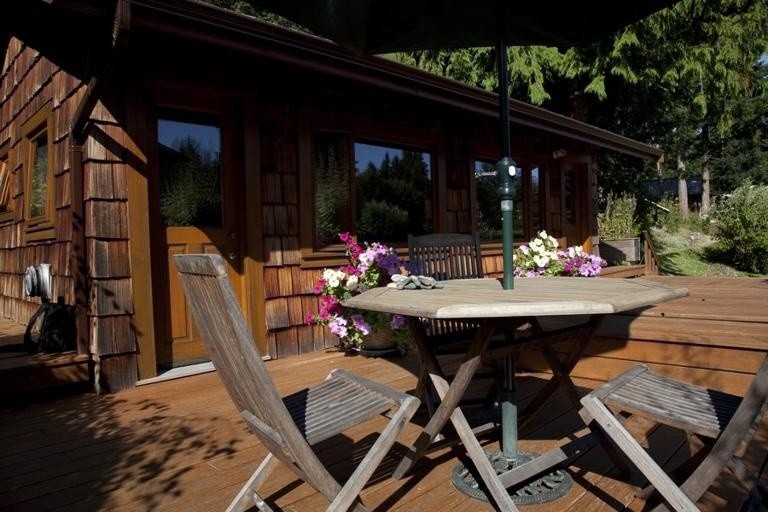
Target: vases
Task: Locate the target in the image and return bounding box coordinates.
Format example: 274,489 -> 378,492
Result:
357,321 -> 396,351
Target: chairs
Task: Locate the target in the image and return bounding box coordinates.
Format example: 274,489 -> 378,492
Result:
571,353 -> 768,512
402,226 -> 503,418
170,251 -> 421,512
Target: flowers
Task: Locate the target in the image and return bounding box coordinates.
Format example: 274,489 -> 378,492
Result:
305,232 -> 425,356
513,229 -> 608,277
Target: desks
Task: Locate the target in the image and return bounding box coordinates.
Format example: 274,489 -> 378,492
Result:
340,278 -> 691,506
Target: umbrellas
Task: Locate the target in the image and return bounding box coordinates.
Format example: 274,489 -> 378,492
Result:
251,1 -> 684,457
